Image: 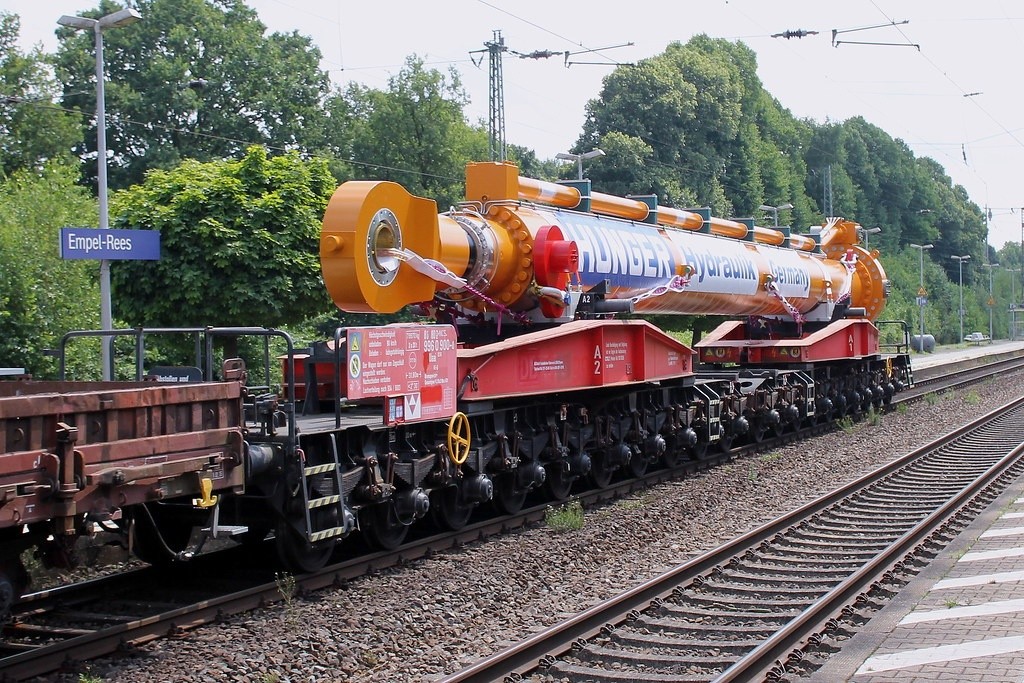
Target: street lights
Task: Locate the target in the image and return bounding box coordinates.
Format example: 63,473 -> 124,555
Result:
54,9 -> 143,383
910,244 -> 934,354
758,204 -> 794,227
1004,268 -> 1021,341
982,263 -> 1000,344
861,227 -> 881,251
951,255 -> 971,343
985,205 -> 992,263
554,149 -> 607,180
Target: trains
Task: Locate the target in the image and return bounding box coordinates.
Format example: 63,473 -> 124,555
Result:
0,160 -> 915,625
